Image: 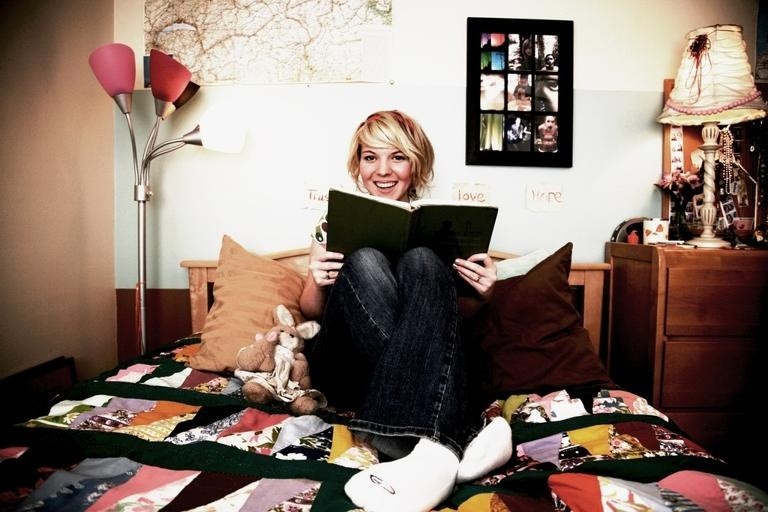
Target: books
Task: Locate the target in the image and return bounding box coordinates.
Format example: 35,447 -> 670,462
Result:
322,187 -> 499,301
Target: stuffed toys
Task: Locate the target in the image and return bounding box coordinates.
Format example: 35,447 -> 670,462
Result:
233,301 -> 329,417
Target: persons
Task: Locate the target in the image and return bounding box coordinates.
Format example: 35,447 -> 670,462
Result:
536,115 -> 558,153
518,37 -> 534,71
540,53 -> 559,72
511,73 -> 531,97
297,106 -> 516,511
534,76 -> 560,113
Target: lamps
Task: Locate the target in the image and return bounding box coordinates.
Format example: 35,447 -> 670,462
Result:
89,44 -> 202,360
654,24 -> 767,249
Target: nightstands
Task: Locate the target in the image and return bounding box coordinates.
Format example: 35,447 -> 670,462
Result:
605,240 -> 767,451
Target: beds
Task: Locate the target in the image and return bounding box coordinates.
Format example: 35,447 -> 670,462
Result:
0,247 -> 766,512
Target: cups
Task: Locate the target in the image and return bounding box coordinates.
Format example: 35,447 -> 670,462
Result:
642,219 -> 670,246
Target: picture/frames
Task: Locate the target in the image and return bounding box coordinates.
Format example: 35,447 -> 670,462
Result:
466,16 -> 572,170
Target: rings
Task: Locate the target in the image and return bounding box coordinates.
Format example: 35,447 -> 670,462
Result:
326,271 -> 330,280
475,275 -> 481,283
